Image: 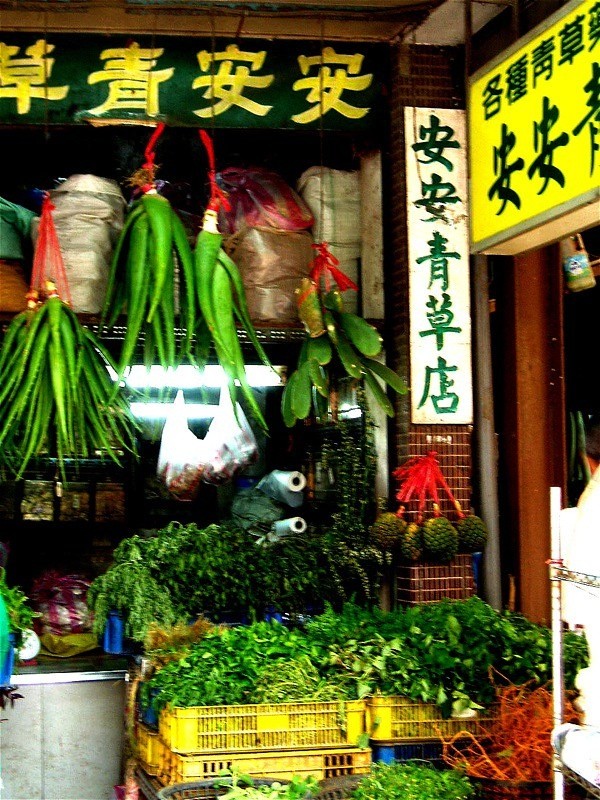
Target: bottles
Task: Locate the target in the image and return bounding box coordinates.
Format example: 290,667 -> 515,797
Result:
560,232 -> 596,292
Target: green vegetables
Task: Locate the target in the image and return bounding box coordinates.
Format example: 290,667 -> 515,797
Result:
82,519 -> 593,800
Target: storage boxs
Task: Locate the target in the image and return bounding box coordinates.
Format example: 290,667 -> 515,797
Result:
126,663 -> 494,793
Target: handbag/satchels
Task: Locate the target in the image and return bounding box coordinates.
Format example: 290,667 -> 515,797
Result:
158,382 -> 256,500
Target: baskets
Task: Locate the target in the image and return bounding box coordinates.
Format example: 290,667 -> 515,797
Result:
126,664 -> 600,799
